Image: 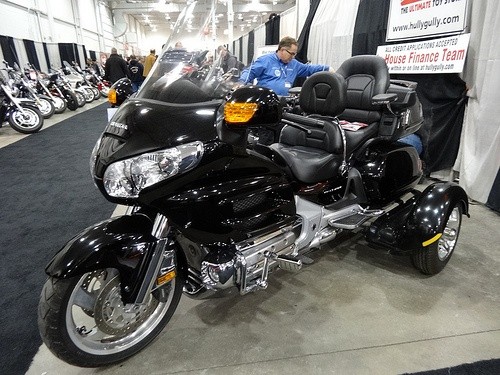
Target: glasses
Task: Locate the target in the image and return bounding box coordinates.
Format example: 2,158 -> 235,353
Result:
285,49 -> 295,56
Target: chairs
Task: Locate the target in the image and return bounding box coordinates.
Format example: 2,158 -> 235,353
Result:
329,54 -> 390,152
270,68 -> 348,184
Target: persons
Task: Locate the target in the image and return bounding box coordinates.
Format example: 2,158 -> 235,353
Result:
143,48 -> 156,81
136,56 -> 146,63
129,55 -> 144,93
106,48 -> 128,87
217,46 -> 244,73
127,57 -> 130,63
234,36 -> 334,96
175,42 -> 182,48
87,58 -> 100,76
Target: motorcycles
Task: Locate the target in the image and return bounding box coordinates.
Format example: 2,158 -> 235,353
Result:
0,59 -> 110,134
36,0 -> 473,368
